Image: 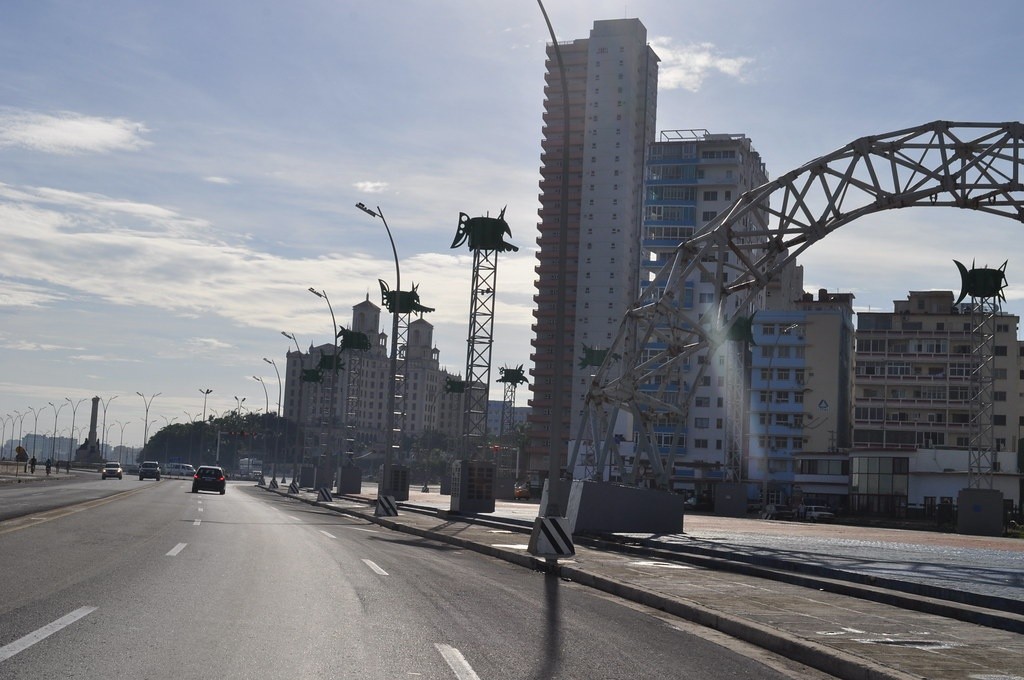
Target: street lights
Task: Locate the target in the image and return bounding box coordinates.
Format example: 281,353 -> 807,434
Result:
264,356 -> 281,491
96,395 -> 118,472
355,202 -> 401,516
49,402 -> 67,458
64,398 -> 87,473
137,392 -> 160,460
199,388 -> 212,465
281,331 -> 303,494
232,396 -> 245,478
28,406 -> 46,459
309,287 -> 337,501
24,405 -> 263,480
0,414 -> 22,461
16,410 -> 31,475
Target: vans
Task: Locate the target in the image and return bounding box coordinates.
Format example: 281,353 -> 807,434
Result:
166,463 -> 196,476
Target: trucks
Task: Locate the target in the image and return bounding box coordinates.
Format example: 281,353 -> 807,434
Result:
254,375 -> 269,487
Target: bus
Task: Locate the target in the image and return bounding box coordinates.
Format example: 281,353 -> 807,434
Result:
240,458 -> 263,471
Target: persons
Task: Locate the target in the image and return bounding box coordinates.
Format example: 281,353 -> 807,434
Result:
56,461 -> 60,474
798,501 -> 806,522
66,462 -> 71,473
44,459 -> 52,473
29,456 -> 37,469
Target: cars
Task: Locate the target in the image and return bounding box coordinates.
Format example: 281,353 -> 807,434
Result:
761,504 -> 793,520
138,461 -> 162,481
804,505 -> 833,523
192,465 -> 226,494
102,462 -> 122,480
